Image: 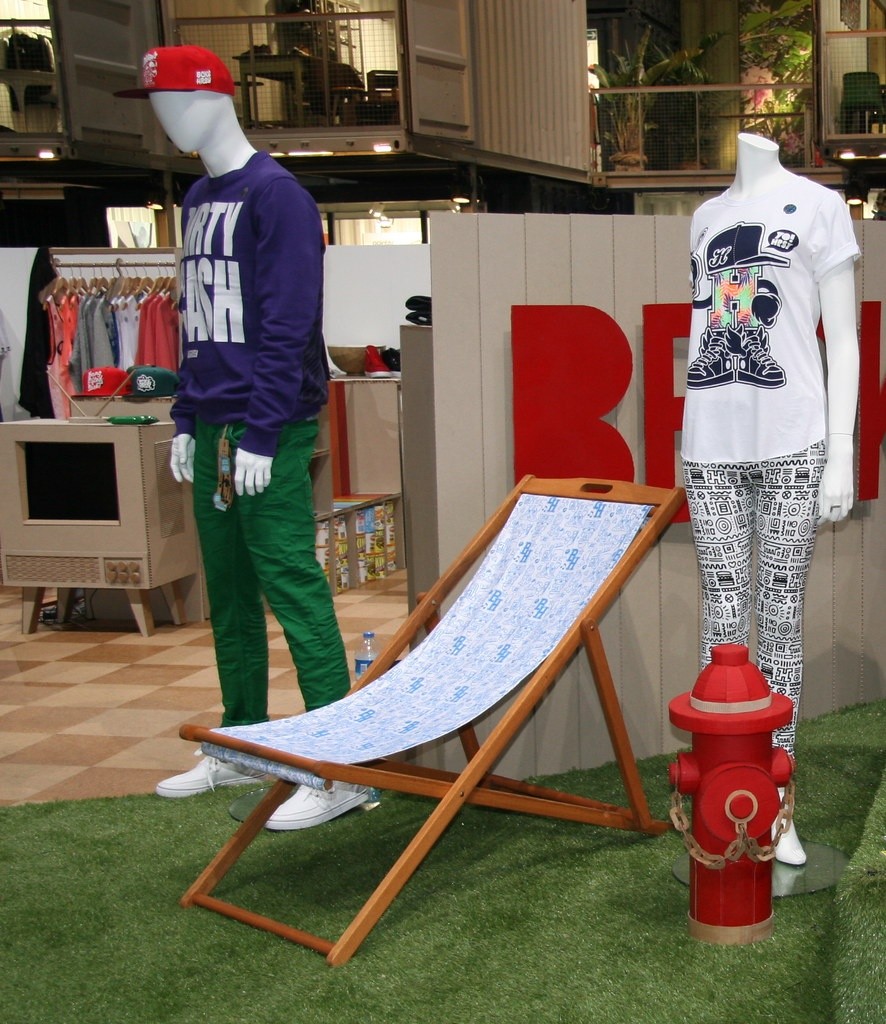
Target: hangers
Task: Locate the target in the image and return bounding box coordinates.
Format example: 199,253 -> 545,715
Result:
38,257 -> 177,310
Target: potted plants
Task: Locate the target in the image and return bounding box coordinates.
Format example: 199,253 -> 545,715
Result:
594,23 -> 708,173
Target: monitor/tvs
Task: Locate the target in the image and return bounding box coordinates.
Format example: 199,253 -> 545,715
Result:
0,370 -> 198,589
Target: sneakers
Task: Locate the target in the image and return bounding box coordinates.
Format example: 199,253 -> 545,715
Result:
156,746 -> 269,798
263,785 -> 370,830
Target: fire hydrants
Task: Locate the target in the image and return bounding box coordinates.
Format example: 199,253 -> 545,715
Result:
671,642 -> 795,945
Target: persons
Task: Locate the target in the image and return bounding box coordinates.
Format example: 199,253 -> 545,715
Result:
142,45 -> 377,831
680,129 -> 862,867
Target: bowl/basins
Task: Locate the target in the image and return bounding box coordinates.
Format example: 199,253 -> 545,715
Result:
327,345 -> 387,375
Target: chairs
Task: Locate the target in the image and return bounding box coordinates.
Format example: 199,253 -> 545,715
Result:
177,475 -> 687,967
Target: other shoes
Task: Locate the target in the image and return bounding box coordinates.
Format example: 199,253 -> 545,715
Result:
364,345 -> 392,377
382,348 -> 401,377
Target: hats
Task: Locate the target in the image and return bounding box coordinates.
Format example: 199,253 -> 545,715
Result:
71,365 -> 132,397
113,45 -> 235,99
123,365 -> 180,397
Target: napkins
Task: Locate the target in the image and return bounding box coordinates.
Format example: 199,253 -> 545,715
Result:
232,54 -> 311,129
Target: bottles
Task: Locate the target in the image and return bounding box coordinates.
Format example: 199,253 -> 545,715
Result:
872,111 -> 880,133
354,631 -> 380,682
